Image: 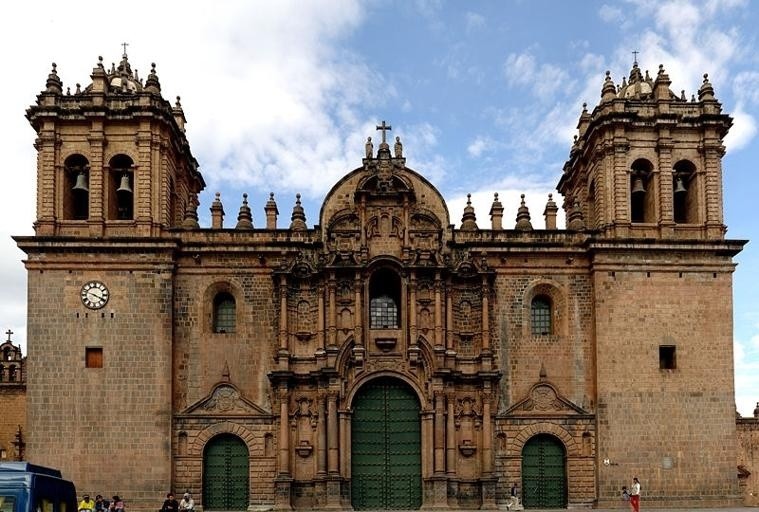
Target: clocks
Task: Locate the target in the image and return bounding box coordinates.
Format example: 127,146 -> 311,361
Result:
79,280 -> 110,311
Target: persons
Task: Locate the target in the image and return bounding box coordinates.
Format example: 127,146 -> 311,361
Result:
179,492 -> 196,512
78,494 -> 125,512
159,493 -> 179,512
506,483 -> 520,511
629,477 -> 641,512
621,487 -> 630,501
